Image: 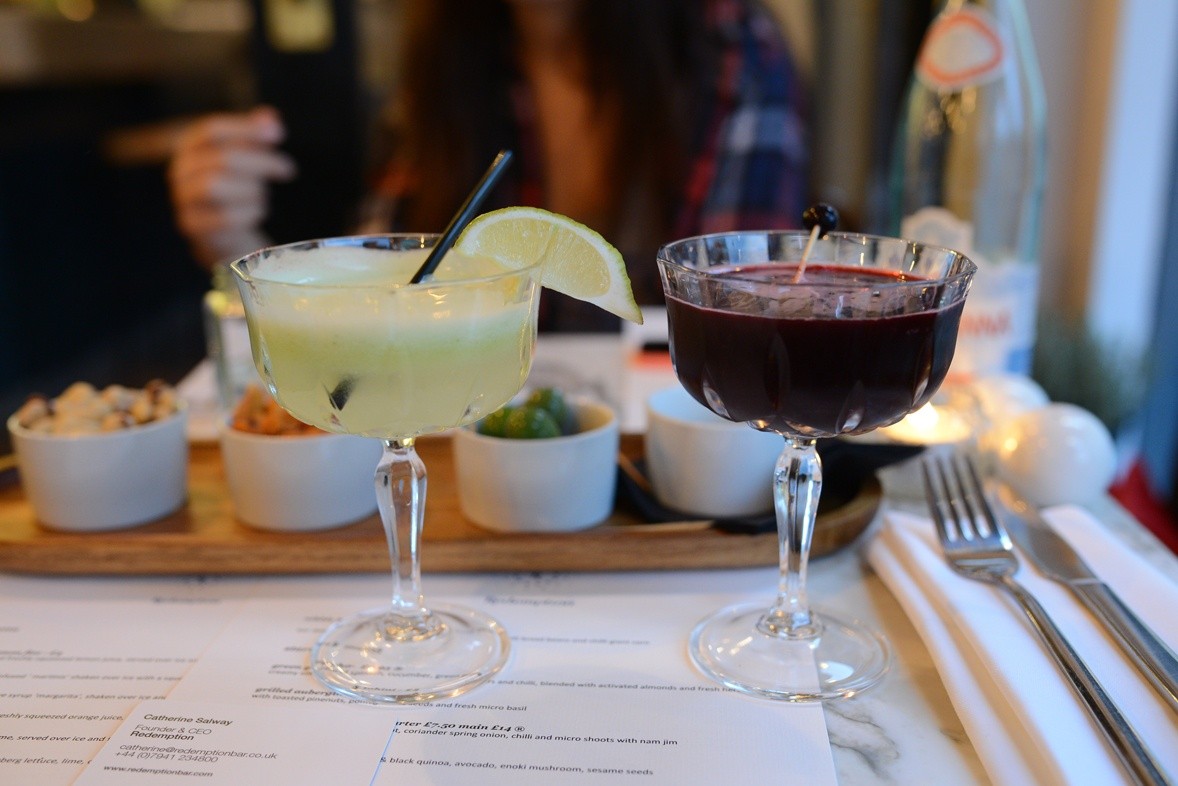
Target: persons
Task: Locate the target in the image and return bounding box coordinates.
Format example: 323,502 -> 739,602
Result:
0,0 -> 1178,516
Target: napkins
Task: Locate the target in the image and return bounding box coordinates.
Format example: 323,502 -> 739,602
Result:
865,504 -> 1178,786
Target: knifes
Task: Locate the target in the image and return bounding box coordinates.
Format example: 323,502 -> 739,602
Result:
982,465 -> 1178,716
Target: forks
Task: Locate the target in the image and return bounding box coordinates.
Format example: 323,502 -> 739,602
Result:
919,451 -> 1168,786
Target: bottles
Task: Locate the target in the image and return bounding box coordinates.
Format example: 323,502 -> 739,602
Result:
203,259 -> 257,405
896,0 -> 1045,393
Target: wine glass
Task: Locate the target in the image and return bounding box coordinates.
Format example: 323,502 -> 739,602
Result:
228,230 -> 547,707
653,228 -> 975,700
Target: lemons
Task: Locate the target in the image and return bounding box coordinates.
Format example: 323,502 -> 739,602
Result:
454,206 -> 643,323
474,384 -> 586,438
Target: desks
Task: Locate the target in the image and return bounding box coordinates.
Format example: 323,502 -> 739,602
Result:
0,311 -> 1178,786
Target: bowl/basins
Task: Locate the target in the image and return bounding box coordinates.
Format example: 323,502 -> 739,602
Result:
450,392 -> 621,532
219,413 -> 379,533
644,386 -> 795,519
6,402 -> 189,533
